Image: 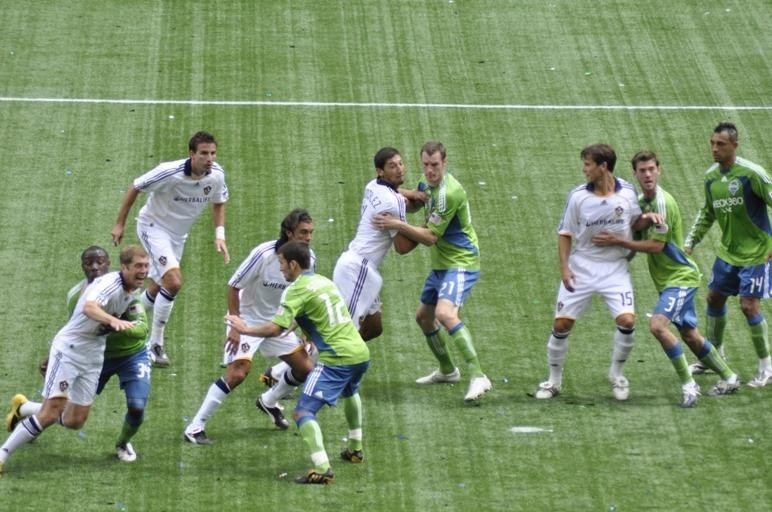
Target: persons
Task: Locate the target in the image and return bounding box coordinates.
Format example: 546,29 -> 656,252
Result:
368,139 -> 495,404
259,146 -> 431,398
39,245 -> 153,466
183,206 -> 317,446
222,239 -> 371,484
0,241 -> 153,476
680,120 -> 772,390
534,142 -> 665,403
593,148 -> 741,408
109,129 -> 233,368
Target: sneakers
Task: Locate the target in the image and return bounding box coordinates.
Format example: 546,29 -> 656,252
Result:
465,375 -> 491,404
184,367 -> 362,483
115,441 -> 136,462
680,358 -> 771,408
147,341 -> 170,367
536,381 -> 560,400
7,394 -> 28,432
610,375 -> 629,401
416,367 -> 460,385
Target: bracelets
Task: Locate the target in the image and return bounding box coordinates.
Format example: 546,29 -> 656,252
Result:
213,225 -> 227,241
